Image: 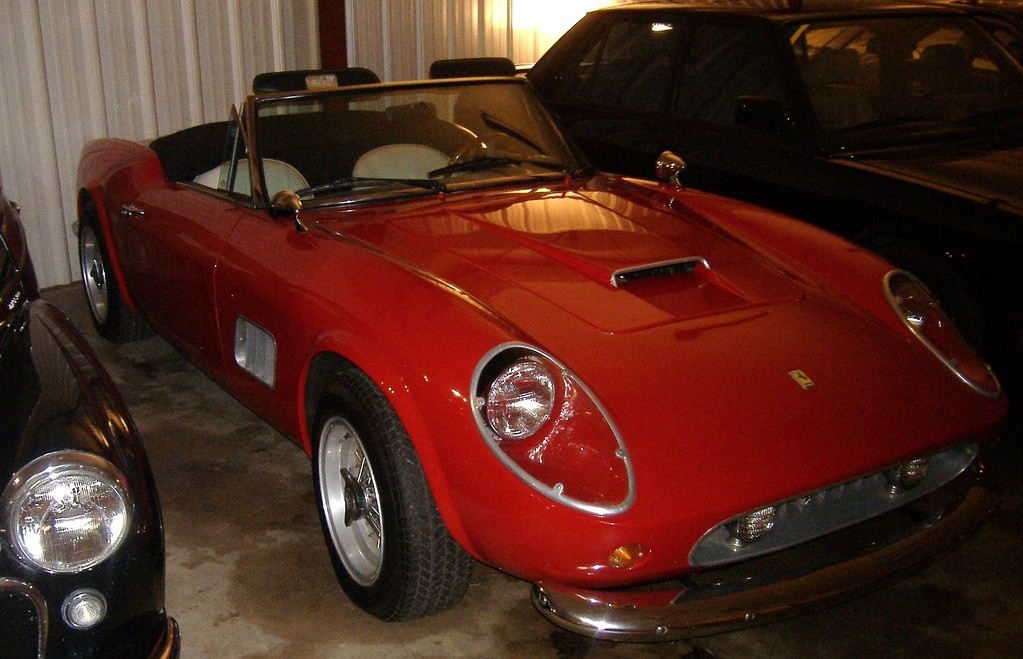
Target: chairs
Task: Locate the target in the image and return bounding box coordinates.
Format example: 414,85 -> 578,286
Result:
903,28 -> 1019,125
196,157 -> 317,205
795,28 -> 915,129
354,144 -> 466,183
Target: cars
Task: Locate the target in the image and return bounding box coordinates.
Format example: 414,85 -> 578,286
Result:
72,54 -> 1013,642
512,0 -> 1023,337
0,167 -> 181,659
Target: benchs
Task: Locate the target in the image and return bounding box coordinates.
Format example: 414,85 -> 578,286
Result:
152,112 -> 476,187
